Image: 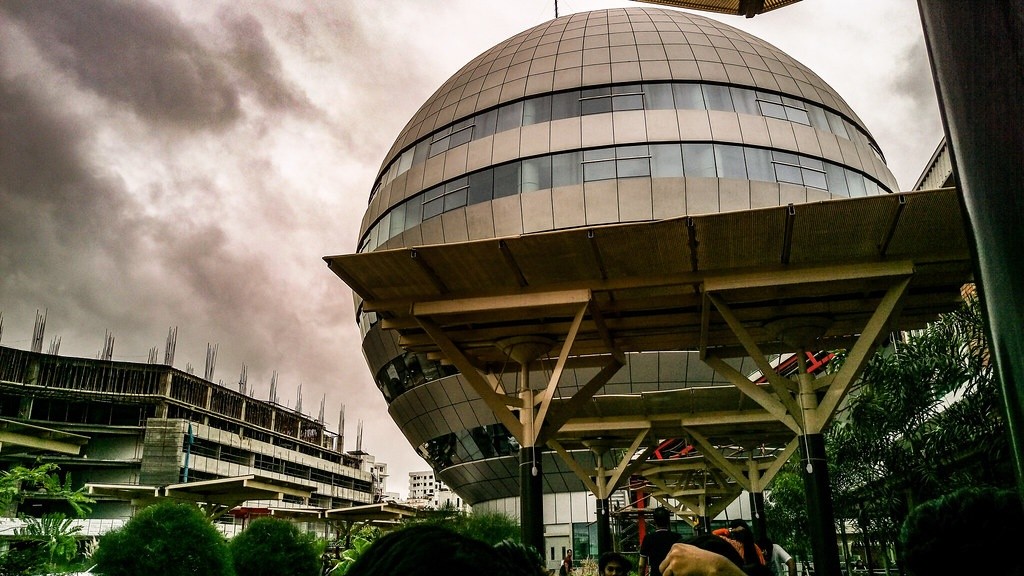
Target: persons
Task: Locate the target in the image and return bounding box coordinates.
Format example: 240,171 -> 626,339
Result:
659,532 -> 748,576
712,519 -> 765,565
638,507 -> 682,576
321,546 -> 344,576
753,528 -> 796,576
564,549 -> 573,576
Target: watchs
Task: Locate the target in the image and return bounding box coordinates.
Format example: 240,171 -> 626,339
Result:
729,528 -> 732,533
